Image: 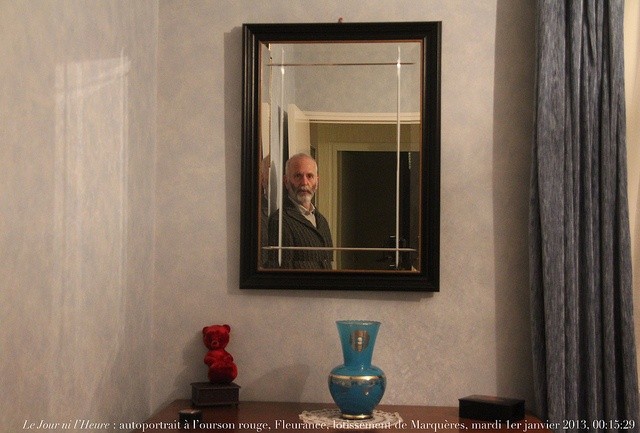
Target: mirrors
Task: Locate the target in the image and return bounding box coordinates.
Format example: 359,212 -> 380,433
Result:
240,23 -> 443,291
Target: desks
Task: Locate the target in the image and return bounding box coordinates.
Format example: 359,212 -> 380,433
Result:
129,400 -> 556,433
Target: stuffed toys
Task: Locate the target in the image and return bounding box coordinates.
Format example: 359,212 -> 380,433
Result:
202,324 -> 238,382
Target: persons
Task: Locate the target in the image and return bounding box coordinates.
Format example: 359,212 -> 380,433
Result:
269,153 -> 333,271
